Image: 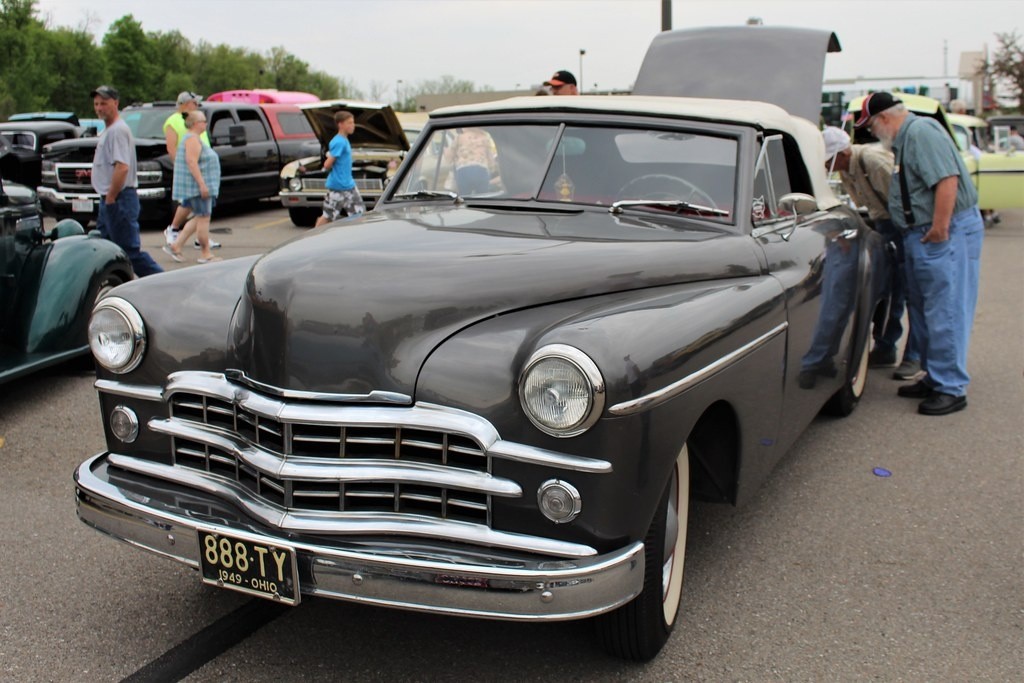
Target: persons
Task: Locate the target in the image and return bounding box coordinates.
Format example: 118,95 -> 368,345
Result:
969,143 -> 1001,225
163,111 -> 222,264
948,99 -> 967,114
1004,125 -> 1024,150
853,93 -> 986,416
543,72 -> 576,96
822,126 -> 923,378
796,217 -> 884,390
446,127 -> 497,195
164,90 -> 221,251
92,86 -> 164,278
315,111 -> 365,228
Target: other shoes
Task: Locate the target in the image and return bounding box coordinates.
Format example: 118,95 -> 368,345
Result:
163,243 -> 185,263
197,254 -> 220,263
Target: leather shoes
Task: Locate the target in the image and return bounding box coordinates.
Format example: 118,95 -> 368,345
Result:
898,376 -> 936,398
918,391 -> 966,414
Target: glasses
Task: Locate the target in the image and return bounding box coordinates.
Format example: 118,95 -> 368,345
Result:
866,114 -> 878,132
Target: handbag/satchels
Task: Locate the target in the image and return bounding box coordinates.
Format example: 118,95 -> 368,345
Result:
444,172 -> 458,193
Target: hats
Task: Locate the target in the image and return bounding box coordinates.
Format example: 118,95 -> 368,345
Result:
91,85 -> 119,100
854,91 -> 903,129
820,126 -> 852,164
542,70 -> 576,87
177,91 -> 202,105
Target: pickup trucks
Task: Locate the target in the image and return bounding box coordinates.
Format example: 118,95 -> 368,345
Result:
35,101 -> 324,235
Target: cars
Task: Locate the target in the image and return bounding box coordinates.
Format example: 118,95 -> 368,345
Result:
845,91 -> 1024,228
73,22 -> 905,666
277,99 -> 459,228
0,93 -> 205,201
0,131 -> 136,389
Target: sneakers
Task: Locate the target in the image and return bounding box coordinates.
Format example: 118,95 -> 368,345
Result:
164,226 -> 179,245
868,351 -> 896,367
894,358 -> 922,379
194,237 -> 221,249
